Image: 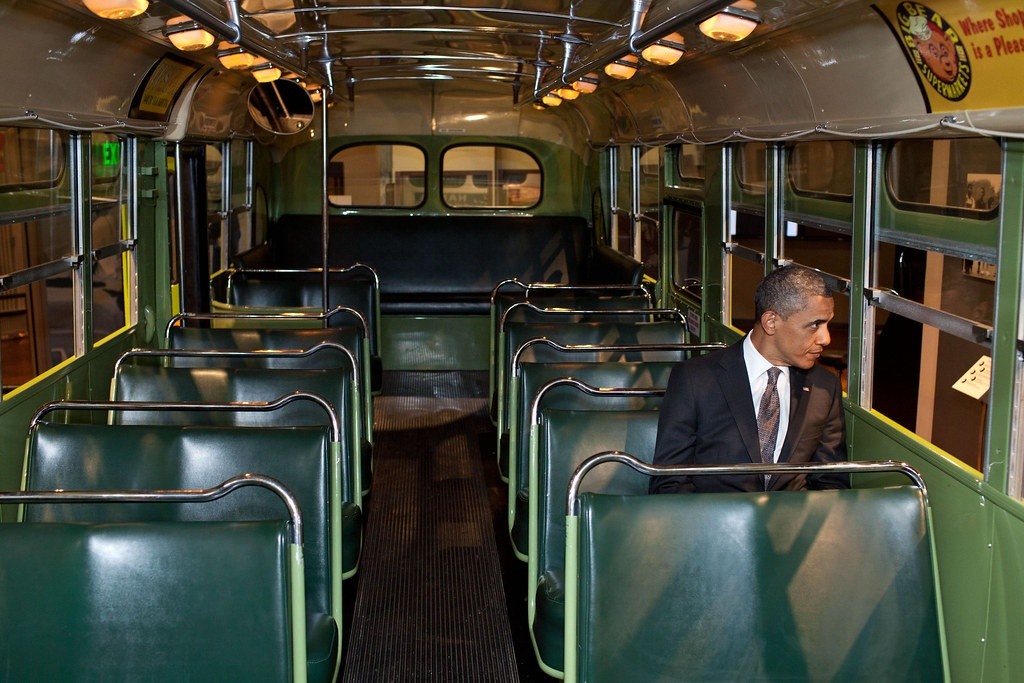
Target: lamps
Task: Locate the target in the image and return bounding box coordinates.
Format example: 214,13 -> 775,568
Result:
80,0 -> 153,19
307,89 -> 322,103
558,86 -> 580,100
572,77 -> 599,94
250,63 -> 282,83
604,59 -> 641,80
695,5 -> 763,41
215,46 -> 258,70
291,78 -> 307,89
542,93 -> 563,106
533,101 -> 548,111
641,39 -> 687,65
162,21 -> 216,51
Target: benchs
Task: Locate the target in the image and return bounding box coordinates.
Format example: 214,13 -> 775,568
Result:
242,215 -> 647,369
1,264 -> 384,683
488,277 -> 952,683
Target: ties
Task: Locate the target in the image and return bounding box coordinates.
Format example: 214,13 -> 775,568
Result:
757,367 -> 784,491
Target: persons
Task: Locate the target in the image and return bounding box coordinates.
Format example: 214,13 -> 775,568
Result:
208,206 -> 241,288
647,264 -> 850,494
91,207 -> 133,314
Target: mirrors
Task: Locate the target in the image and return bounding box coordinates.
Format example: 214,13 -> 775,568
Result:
246,78 -> 315,135
